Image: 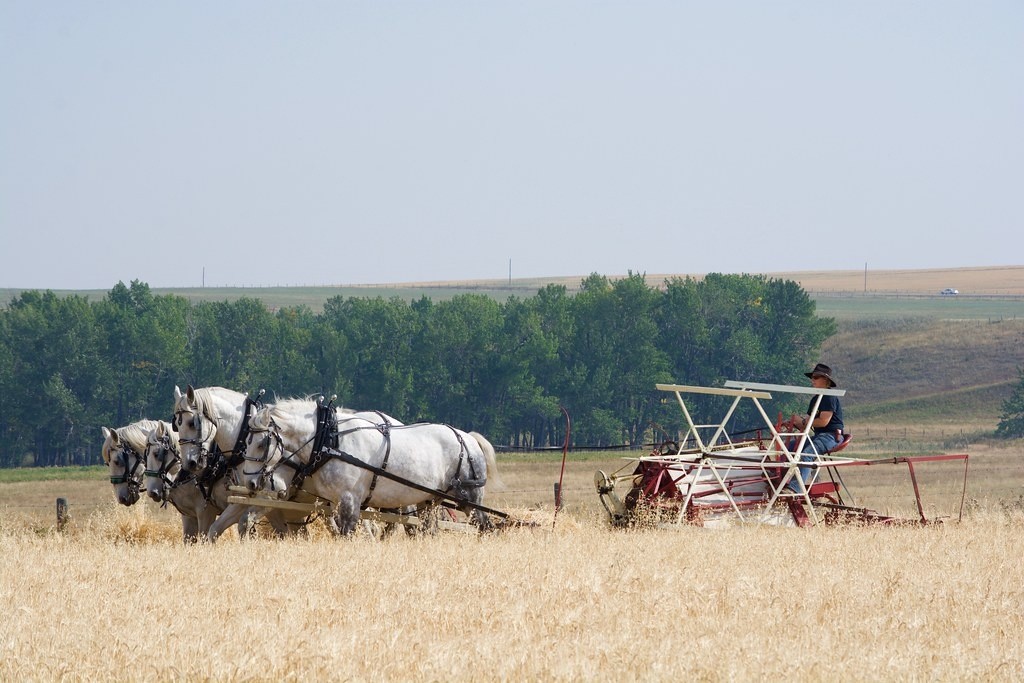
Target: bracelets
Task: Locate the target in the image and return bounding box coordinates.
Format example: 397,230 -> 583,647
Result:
800,416 -> 806,426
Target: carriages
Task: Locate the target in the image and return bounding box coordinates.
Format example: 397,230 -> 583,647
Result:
101,382 -> 970,545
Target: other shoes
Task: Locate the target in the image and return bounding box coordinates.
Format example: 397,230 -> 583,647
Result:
781,489 -> 800,500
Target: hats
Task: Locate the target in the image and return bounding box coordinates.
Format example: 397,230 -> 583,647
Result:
804,363 -> 837,388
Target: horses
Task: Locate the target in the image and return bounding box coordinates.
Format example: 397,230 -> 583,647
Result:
101,385 -> 505,546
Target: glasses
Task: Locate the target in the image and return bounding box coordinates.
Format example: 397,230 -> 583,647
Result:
809,375 -> 825,380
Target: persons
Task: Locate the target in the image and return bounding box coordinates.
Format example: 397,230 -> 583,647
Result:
780,363 -> 844,498
